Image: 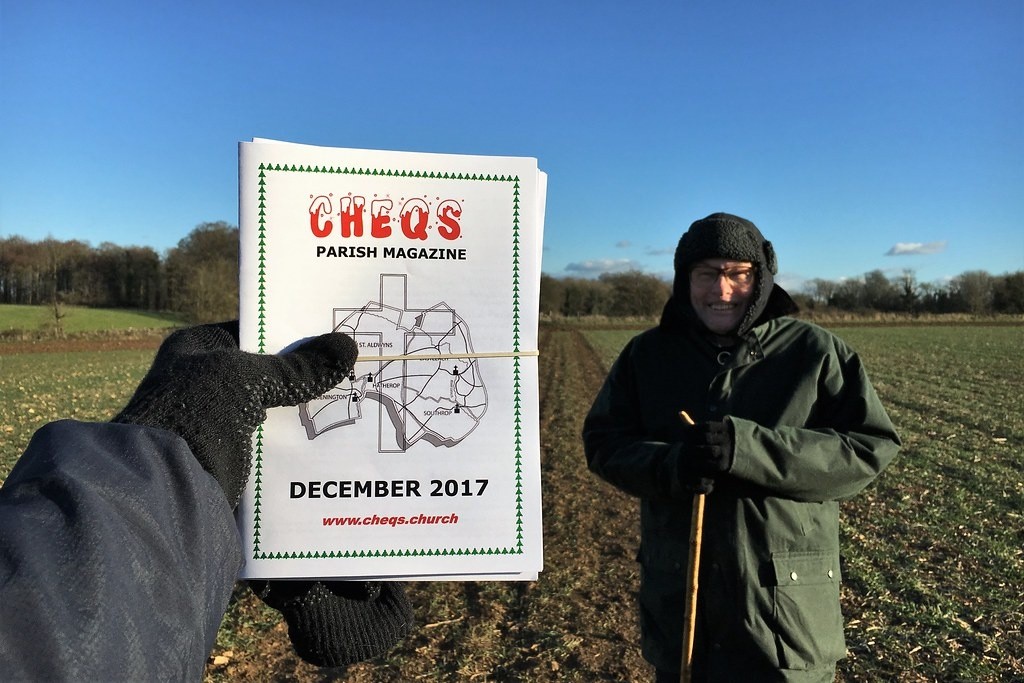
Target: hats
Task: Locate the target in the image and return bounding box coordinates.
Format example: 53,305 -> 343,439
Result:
674,212 -> 777,337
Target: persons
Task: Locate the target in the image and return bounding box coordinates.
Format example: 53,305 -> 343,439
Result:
580,211 -> 899,681
0,321 -> 357,683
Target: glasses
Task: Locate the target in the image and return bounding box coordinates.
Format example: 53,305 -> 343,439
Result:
689,263 -> 755,287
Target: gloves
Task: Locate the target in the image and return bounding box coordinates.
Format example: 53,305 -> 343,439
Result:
107,319 -> 359,512
673,420 -> 732,494
247,580 -> 416,669
